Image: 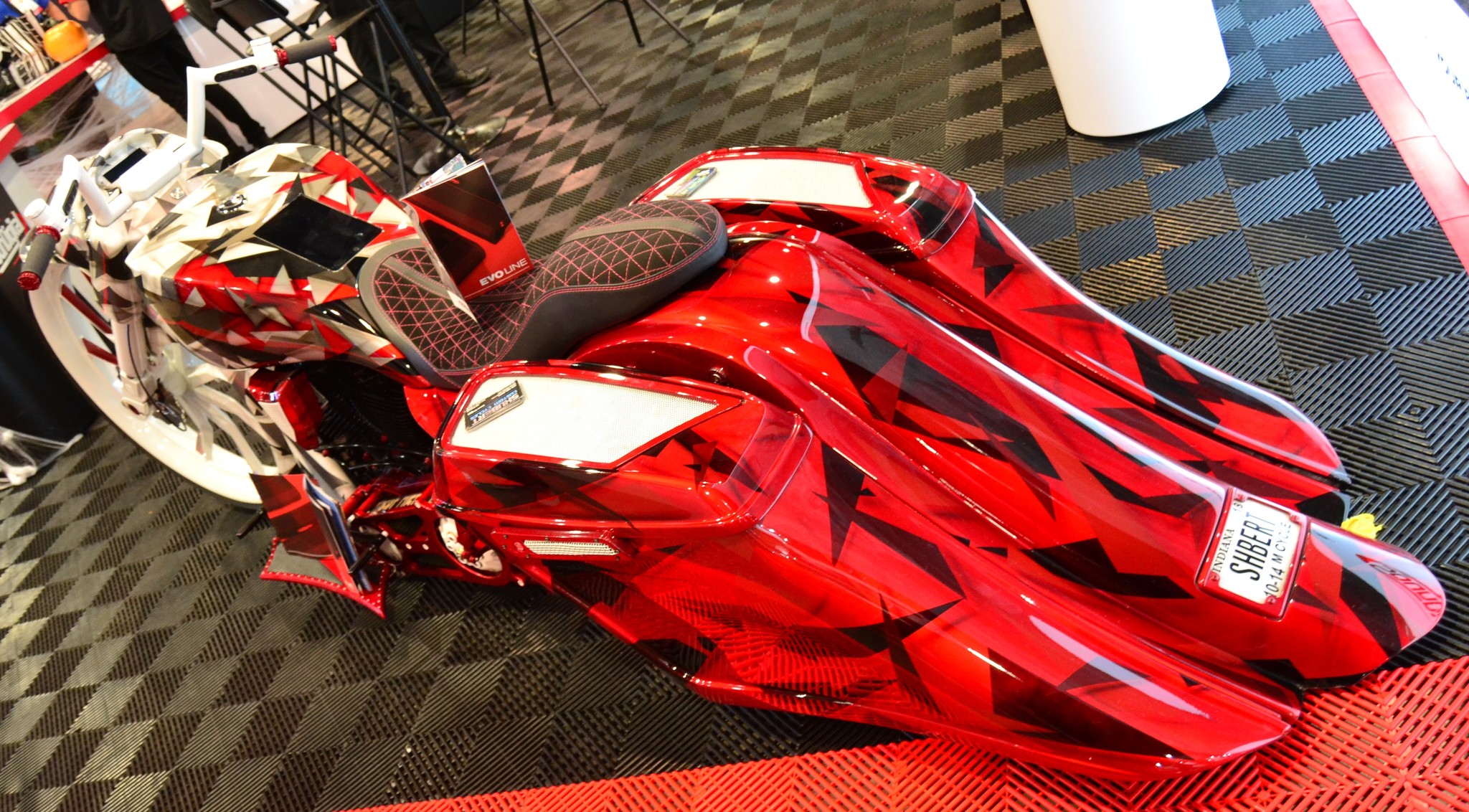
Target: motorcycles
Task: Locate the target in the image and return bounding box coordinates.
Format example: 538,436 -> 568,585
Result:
17,36 -> 1445,786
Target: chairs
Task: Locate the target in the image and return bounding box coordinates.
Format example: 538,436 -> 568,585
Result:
183,0 -> 453,182
212,1 -> 478,196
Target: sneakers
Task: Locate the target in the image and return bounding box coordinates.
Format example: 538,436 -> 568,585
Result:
440,66 -> 491,91
400,101 -> 432,125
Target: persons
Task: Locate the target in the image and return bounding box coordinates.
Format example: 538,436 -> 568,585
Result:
319,0 -> 493,121
41,1 -> 274,165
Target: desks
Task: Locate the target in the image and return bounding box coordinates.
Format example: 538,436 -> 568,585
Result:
0,5 -> 188,481
